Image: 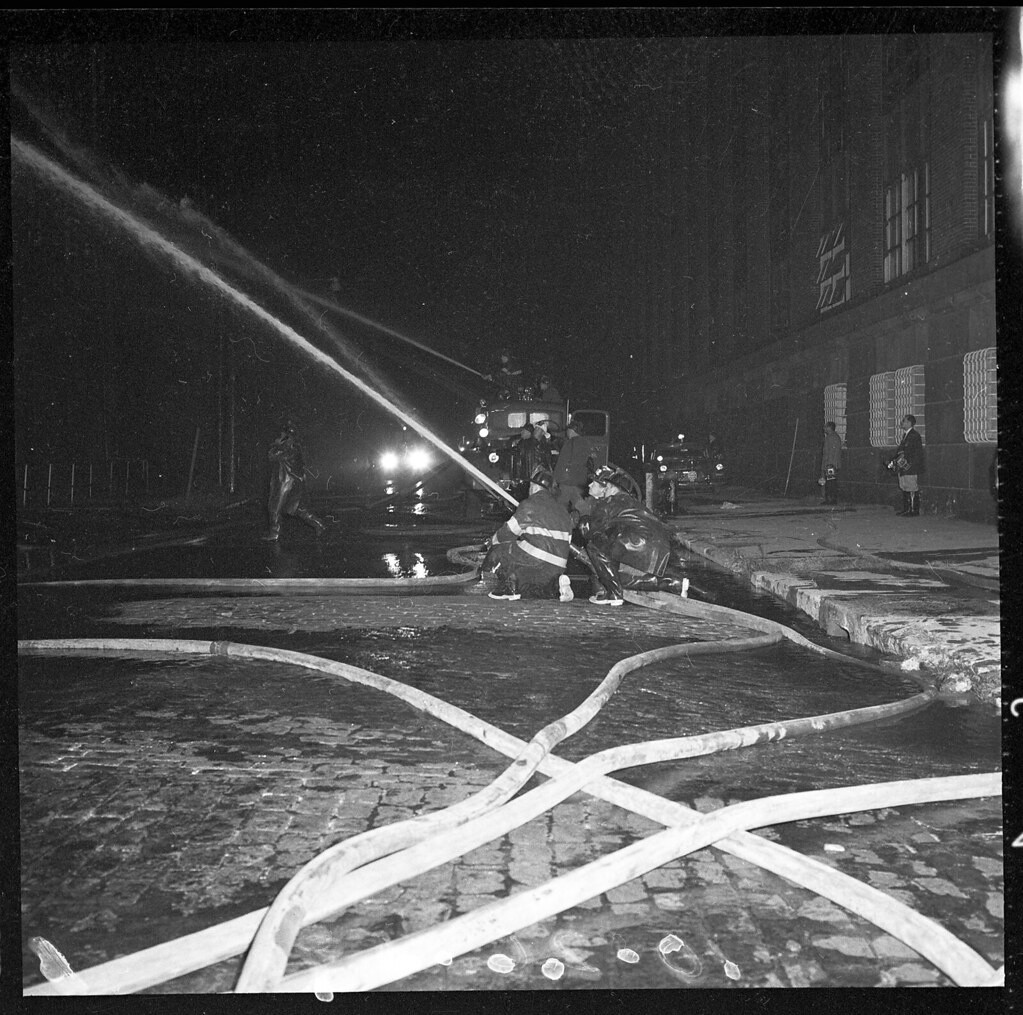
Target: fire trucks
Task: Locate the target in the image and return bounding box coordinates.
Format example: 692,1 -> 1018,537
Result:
456,389 -> 611,517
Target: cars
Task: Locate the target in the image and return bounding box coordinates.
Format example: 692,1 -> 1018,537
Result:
649,438 -> 731,503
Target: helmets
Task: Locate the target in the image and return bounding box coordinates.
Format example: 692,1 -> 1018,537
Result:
604,472 -> 632,494
567,420 -> 583,436
520,423 -> 534,433
588,465 -> 614,485
530,470 -> 554,491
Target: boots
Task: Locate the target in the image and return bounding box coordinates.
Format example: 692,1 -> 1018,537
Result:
259,512 -> 282,542
308,514 -> 329,537
821,479 -> 838,506
897,490 -> 920,517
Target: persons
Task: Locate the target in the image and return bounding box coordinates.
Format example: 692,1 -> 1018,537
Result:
260,423 -> 327,542
513,422 -> 552,499
820,422 -> 841,507
572,469 -> 689,606
484,471 -> 574,604
702,431 -> 723,458
892,415 -> 925,518
553,422 -> 587,509
482,350 -> 565,404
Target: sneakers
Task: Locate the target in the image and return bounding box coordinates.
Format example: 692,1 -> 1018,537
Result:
557,574 -> 574,603
669,578 -> 690,599
589,593 -> 624,607
489,586 -> 520,602
